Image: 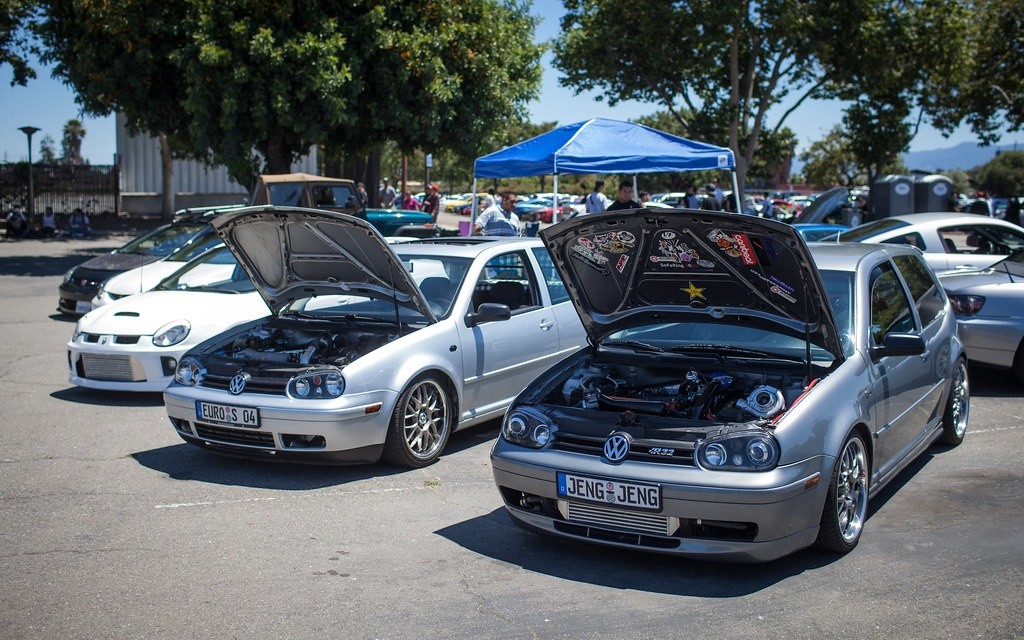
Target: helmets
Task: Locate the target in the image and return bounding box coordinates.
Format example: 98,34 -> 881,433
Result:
432,184 -> 439,192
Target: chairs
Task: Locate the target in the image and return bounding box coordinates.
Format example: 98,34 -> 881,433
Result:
419,277 -> 452,308
483,282 -> 527,311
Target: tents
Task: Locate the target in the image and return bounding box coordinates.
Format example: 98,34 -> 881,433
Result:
467,117 -> 742,236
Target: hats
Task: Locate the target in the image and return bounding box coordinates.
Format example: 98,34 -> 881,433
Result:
706,183 -> 717,195
383,176 -> 389,183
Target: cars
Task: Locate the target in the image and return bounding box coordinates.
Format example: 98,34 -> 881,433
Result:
791,224 -> 852,243
440,193 -> 572,224
490,202 -> 971,559
163,204 -> 592,469
57,171 -> 441,315
90,224 -> 238,312
752,189 -> 819,217
817,211 -> 1024,272
934,248 -> 1023,390
66,237 -> 451,392
650,193 -> 688,207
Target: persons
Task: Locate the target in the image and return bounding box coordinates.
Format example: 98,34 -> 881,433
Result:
5,204 -> 29,239
63,208 -> 91,238
355,177 -> 439,218
949,192 -> 963,211
606,181 -> 642,211
977,191 -> 995,216
639,189 -> 649,207
585,179 -> 607,213
744,192 -> 773,218
36,206 -> 60,238
676,180 -> 741,213
472,189 -> 523,237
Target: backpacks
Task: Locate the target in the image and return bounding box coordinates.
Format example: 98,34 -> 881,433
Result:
8,211 -> 25,233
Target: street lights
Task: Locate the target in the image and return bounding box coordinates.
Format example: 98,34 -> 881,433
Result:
17,127 -> 42,218
